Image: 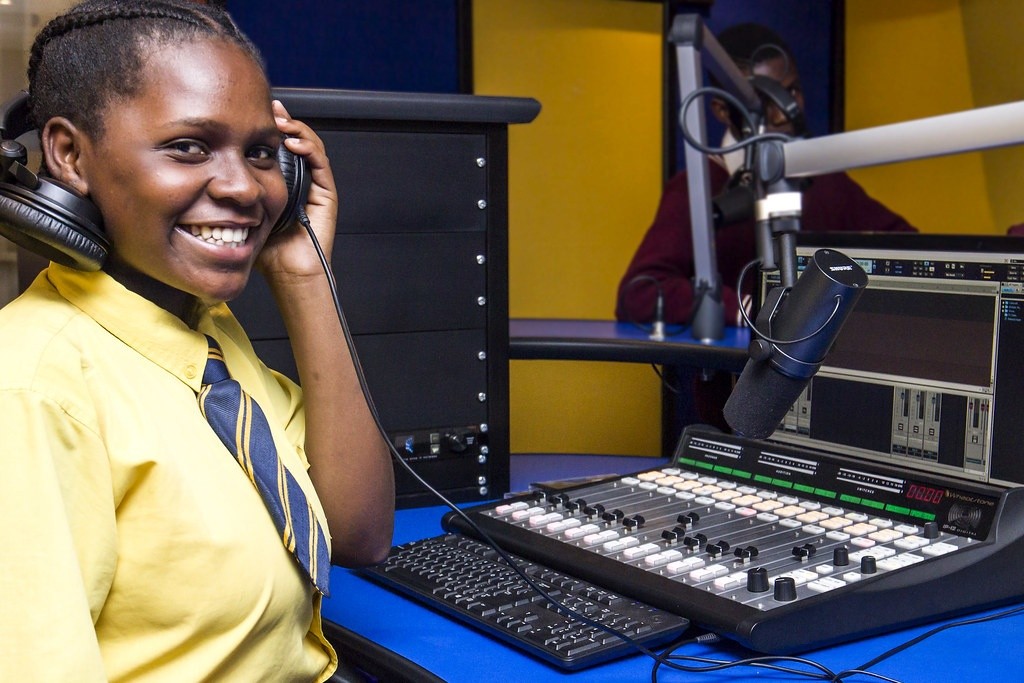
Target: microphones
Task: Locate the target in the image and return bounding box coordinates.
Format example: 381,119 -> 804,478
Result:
723,248 -> 870,439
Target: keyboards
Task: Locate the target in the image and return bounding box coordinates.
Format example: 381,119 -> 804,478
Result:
354,531 -> 689,672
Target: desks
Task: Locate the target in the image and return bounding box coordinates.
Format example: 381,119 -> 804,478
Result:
308,451 -> 1024,682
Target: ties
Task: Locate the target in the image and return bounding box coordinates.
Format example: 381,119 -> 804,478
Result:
196,335 -> 330,599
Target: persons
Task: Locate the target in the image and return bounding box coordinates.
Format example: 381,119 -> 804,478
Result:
614,22 -> 1024,436
0,0 -> 396,683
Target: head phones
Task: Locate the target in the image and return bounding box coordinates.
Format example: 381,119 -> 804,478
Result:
0,88 -> 314,275
